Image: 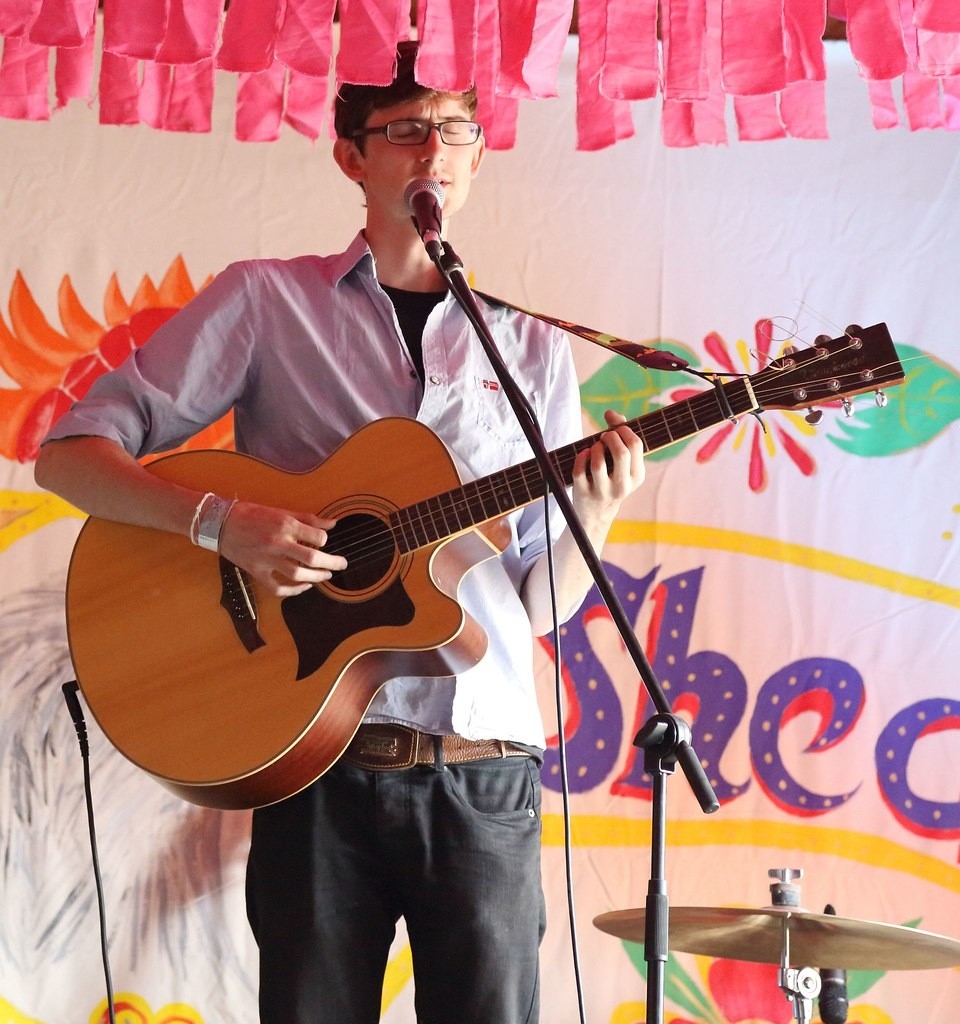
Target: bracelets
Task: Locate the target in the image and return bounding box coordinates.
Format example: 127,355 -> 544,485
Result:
190,492 -> 239,552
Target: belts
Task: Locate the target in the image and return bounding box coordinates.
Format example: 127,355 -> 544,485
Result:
342,723 -> 531,771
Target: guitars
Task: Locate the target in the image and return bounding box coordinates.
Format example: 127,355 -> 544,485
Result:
65,319 -> 907,811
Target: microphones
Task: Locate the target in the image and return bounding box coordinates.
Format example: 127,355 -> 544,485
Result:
818,904 -> 847,1024
402,177 -> 446,262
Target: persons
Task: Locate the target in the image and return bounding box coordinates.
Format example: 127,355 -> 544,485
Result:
35,41 -> 645,1024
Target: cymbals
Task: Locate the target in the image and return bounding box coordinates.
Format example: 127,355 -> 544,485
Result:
591,903 -> 960,974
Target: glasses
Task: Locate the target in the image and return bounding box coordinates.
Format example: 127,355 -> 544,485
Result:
352,119 -> 483,146
